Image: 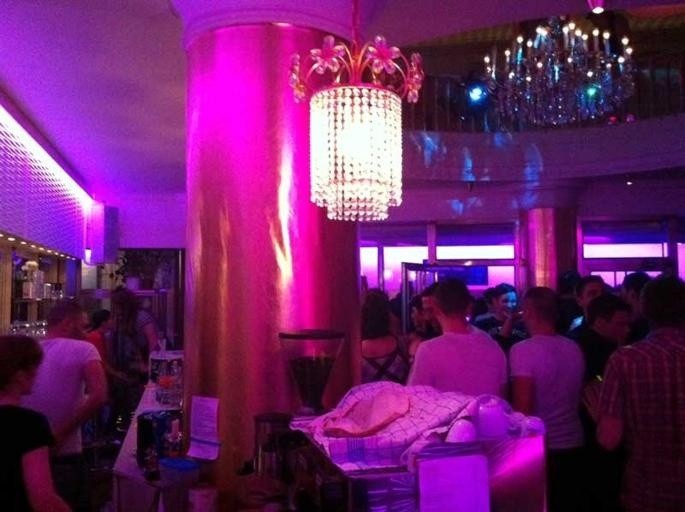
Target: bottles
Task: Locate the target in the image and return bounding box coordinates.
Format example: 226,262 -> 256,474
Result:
23,270 -> 32,298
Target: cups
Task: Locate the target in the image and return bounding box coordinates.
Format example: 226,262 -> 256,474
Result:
444,399 -> 544,444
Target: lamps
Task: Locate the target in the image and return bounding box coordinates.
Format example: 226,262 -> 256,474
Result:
483,14 -> 635,128
288,0 -> 426,222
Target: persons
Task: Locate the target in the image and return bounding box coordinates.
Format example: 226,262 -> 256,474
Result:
364,274 -> 684,512
1,274 -> 158,511
